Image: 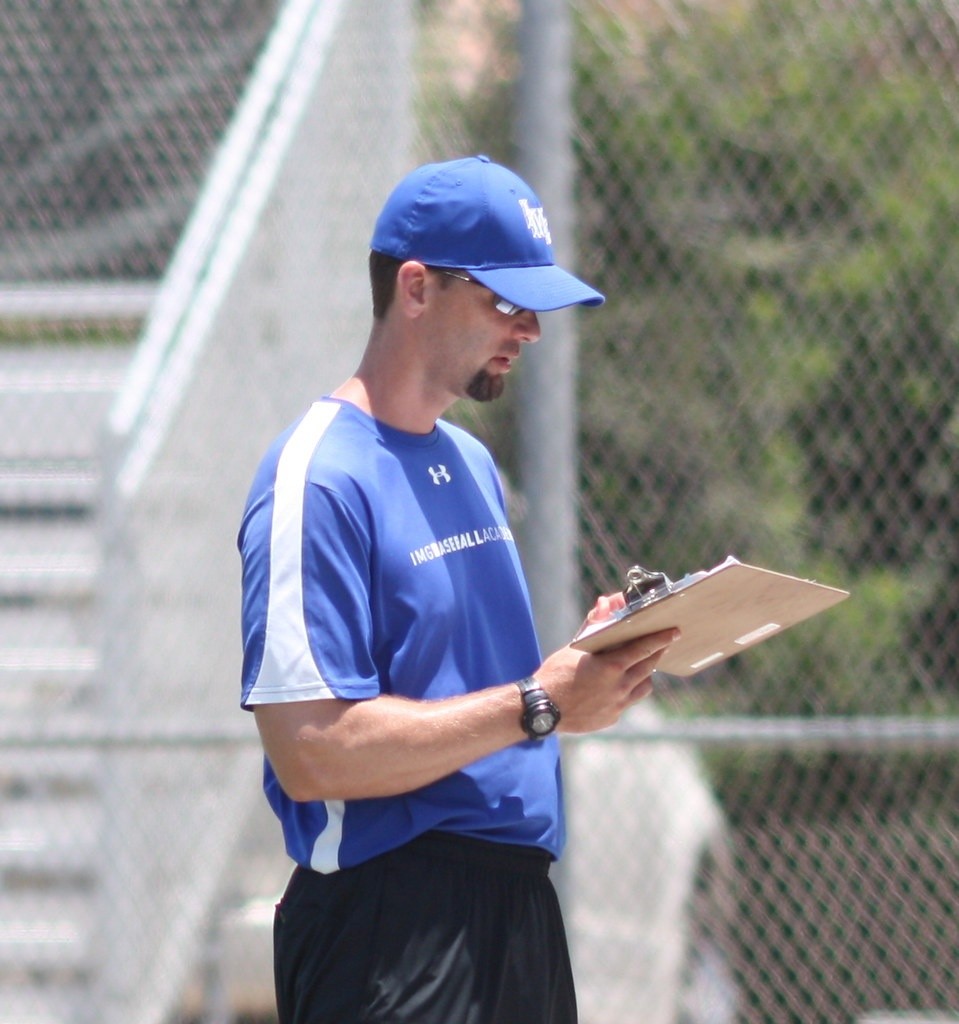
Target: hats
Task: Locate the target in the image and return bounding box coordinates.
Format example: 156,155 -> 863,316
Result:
371,153 -> 608,310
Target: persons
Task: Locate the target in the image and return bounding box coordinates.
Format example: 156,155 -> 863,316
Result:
239,154 -> 680,1024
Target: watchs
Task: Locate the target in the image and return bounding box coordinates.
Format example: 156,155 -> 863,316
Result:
516,677 -> 559,742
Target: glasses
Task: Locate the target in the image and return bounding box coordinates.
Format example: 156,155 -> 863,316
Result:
440,269 -> 526,317
